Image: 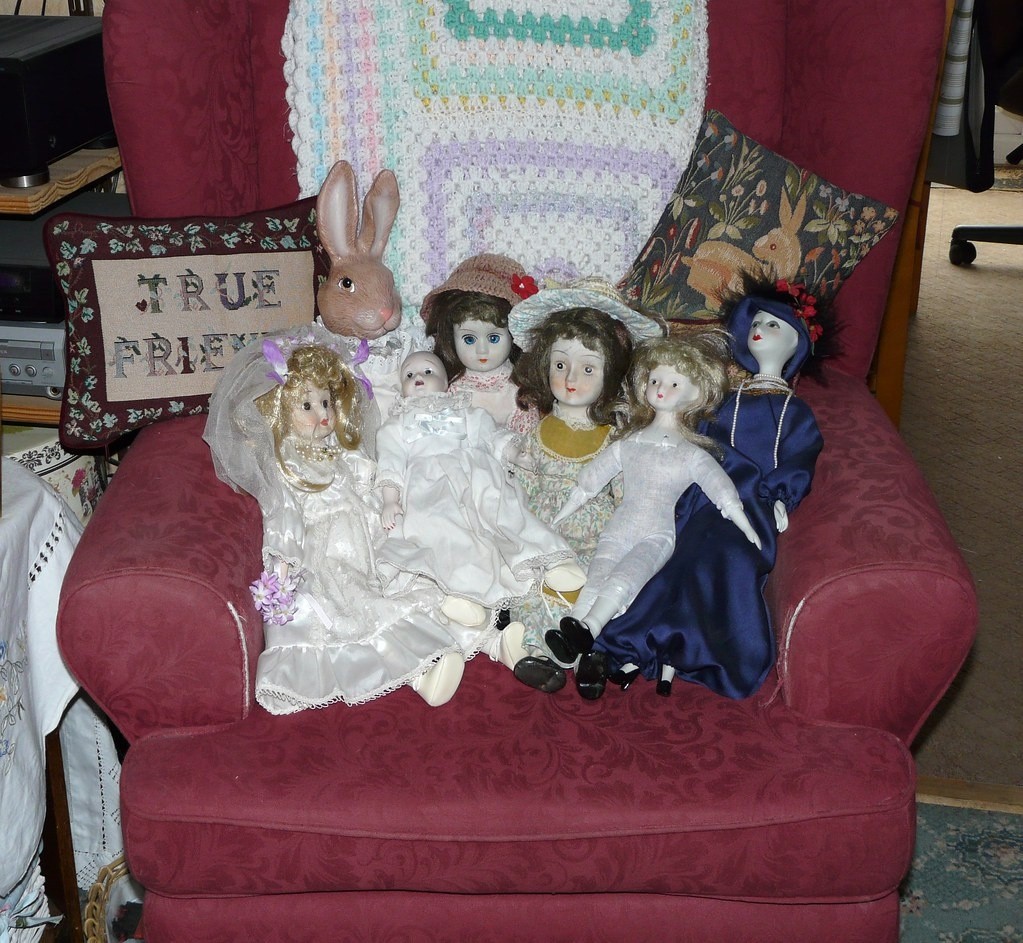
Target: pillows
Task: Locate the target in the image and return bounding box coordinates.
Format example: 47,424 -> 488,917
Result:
622,111 -> 903,321
45,195 -> 333,459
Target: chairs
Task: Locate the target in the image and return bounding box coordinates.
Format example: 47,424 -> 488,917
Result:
948,0 -> 1023,268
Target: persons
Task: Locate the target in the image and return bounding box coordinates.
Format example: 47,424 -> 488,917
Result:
596,284 -> 824,702
206,253 -> 665,716
543,329 -> 764,664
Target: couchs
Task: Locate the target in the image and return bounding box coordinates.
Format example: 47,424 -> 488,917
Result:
56,0 -> 981,943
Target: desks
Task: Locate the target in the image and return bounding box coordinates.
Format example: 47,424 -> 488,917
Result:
0,148 -> 124,426
